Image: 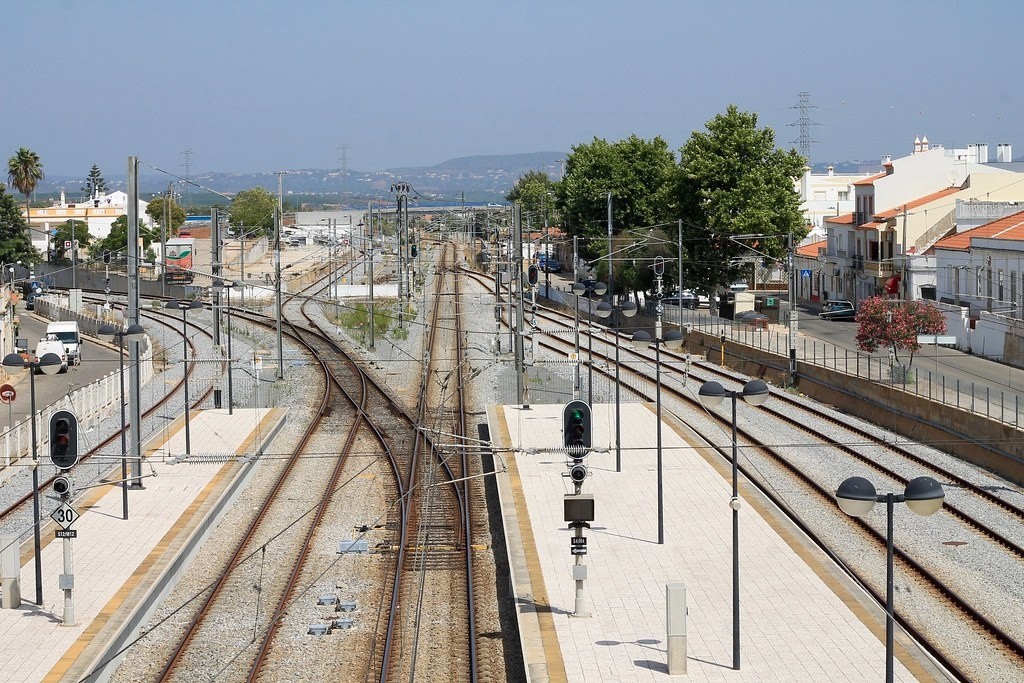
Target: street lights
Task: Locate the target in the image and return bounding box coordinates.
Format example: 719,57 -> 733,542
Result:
632,329 -> 684,544
165,301 -> 204,458
152,191 -> 183,274
2,353 -> 62,606
528,265 -> 538,285
1,260 -> 14,285
572,282 -> 607,410
835,476 -> 946,683
698,380 -> 770,669
596,302 -> 638,472
97,324 -> 145,520
212,280 -> 244,415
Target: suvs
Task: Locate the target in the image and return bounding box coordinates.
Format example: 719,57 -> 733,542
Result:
33,338 -> 68,375
661,293 -> 700,309
822,299 -> 855,321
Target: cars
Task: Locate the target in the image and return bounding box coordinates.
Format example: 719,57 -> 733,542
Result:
534,251 -> 549,261
27,293 -> 41,308
540,260 -> 562,273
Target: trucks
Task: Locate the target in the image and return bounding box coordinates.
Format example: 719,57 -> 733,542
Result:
46,321 -> 83,365
164,237 -> 197,285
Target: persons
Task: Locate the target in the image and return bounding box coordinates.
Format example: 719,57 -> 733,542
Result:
536,257 -> 539,270
588,275 -> 593,283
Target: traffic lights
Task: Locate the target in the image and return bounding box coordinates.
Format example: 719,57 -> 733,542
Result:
49,409 -> 78,469
562,399 -> 592,460
411,245 -> 417,257
654,256 -> 664,275
103,249 -> 111,264
